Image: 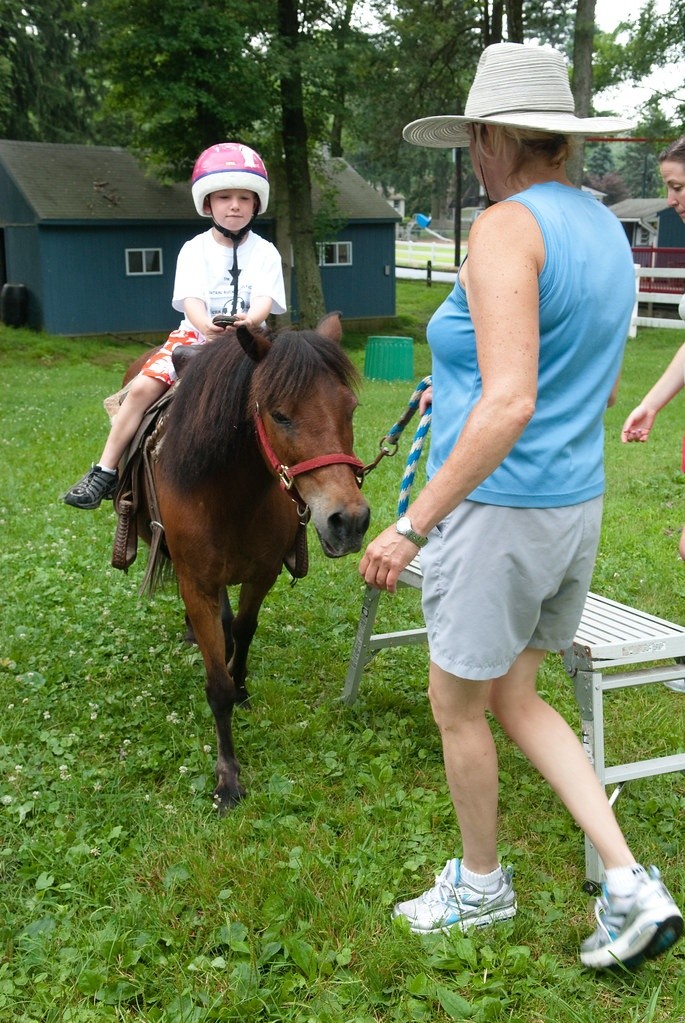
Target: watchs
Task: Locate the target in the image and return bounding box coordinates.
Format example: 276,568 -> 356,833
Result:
396,517 -> 429,547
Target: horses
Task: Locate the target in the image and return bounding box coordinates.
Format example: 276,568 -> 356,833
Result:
121,313 -> 371,811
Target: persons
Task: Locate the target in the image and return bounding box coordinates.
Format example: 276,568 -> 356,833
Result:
64,143 -> 287,510
621,134 -> 685,694
360,42 -> 684,977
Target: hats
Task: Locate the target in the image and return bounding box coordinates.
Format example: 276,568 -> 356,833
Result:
402,42 -> 638,148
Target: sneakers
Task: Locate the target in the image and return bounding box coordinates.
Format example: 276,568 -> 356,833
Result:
392,858 -> 518,934
580,865 -> 684,970
64,464 -> 119,508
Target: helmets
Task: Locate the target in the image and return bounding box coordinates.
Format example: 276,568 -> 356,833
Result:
192,143 -> 269,217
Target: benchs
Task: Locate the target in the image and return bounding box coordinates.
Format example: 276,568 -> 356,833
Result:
341,548 -> 685,894
